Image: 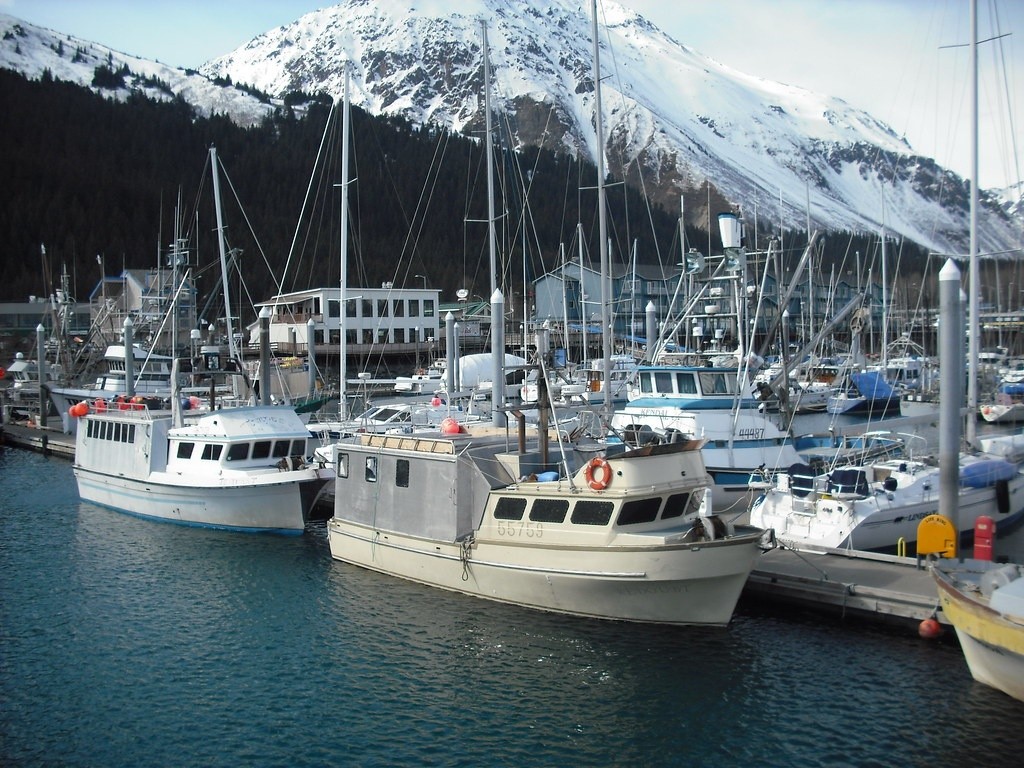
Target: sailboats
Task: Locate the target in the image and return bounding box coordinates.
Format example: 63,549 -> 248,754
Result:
1,0 -> 1024,702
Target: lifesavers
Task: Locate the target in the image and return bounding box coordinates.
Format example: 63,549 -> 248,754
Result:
585,457 -> 612,491
